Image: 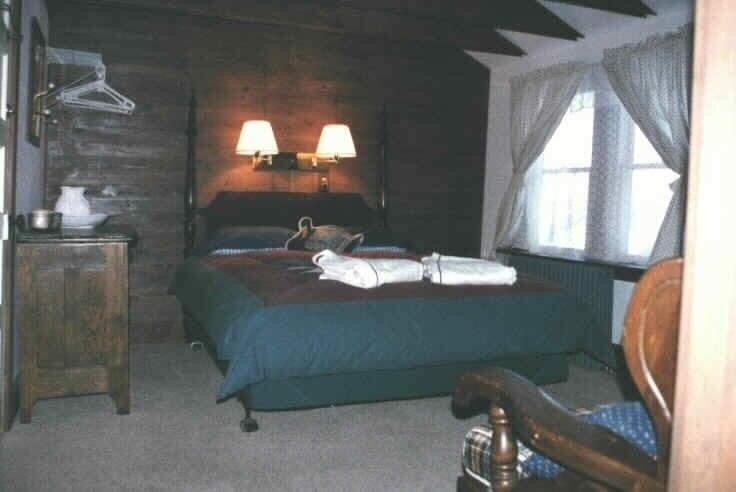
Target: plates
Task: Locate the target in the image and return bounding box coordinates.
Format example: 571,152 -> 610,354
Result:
61,213 -> 109,229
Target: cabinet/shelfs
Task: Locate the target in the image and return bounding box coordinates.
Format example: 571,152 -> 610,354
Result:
13,225 -> 138,423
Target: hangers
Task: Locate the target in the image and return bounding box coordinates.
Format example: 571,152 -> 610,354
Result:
60,64 -> 136,116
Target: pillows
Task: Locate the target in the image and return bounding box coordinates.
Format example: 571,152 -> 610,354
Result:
520,400 -> 655,479
205,216 -> 408,257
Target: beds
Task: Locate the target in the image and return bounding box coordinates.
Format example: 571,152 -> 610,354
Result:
169,89 -> 585,432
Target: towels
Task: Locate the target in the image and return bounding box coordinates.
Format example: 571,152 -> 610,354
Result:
310,246 -> 519,289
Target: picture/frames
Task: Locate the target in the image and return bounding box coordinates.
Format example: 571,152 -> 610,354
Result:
25,15 -> 45,148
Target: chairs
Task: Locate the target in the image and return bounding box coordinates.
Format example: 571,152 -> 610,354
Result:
451,258 -> 683,491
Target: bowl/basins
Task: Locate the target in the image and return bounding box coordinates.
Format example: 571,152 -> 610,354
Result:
28,208 -> 60,232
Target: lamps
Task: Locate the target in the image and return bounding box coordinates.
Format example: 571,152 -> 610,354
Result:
236,120 -> 357,173
